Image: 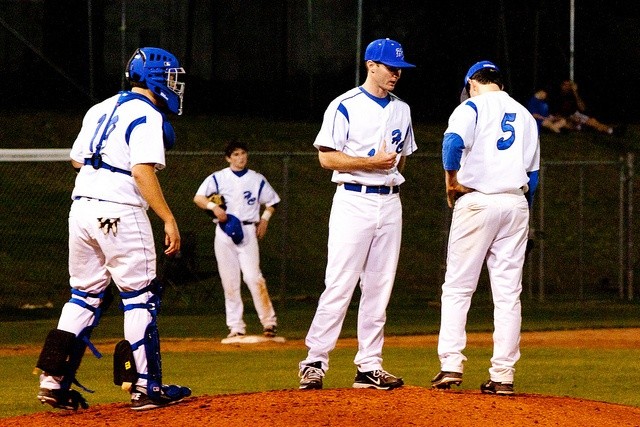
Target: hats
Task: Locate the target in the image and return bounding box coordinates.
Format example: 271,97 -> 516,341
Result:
364,37 -> 416,68
460,60 -> 500,103
219,214 -> 244,245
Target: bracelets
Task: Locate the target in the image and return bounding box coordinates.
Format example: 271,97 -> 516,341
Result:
206,201 -> 216,210
261,210 -> 272,221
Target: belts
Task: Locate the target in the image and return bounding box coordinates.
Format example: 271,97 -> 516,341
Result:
242,221 -> 253,225
343,182 -> 401,195
75,196 -> 105,201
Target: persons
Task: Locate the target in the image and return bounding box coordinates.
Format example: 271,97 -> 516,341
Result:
299,38 -> 419,390
192,140 -> 282,340
528,87 -> 566,133
430,59 -> 541,396
37,46 -> 190,410
555,80 -> 622,133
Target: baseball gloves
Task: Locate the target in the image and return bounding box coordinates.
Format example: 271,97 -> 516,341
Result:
204,193 -> 227,218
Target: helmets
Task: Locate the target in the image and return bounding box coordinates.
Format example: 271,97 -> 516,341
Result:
125,47 -> 186,116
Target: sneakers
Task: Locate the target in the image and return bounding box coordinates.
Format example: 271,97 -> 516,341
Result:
36,388 -> 89,412
130,382 -> 192,411
263,324 -> 278,337
351,366 -> 404,390
226,332 -> 245,338
431,370 -> 463,390
480,378 -> 515,394
297,361 -> 326,390
